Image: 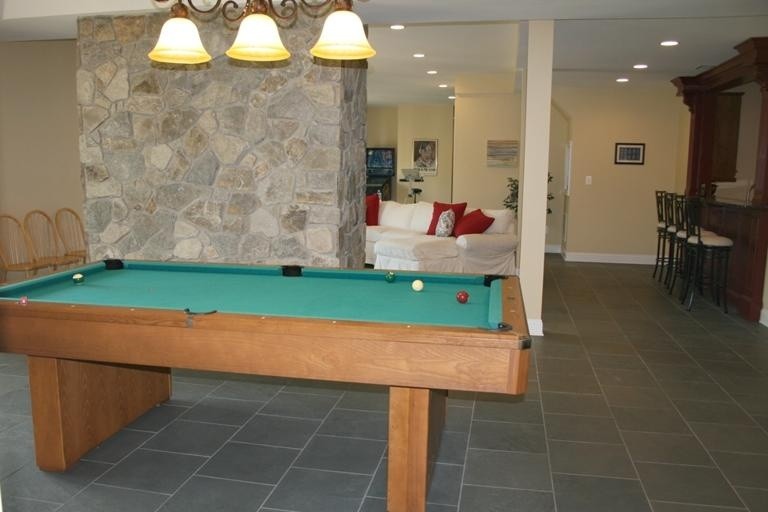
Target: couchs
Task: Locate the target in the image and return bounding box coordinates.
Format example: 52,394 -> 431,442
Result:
365,201 -> 520,275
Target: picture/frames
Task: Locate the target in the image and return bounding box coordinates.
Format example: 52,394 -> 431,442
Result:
412,138 -> 438,176
614,142 -> 645,165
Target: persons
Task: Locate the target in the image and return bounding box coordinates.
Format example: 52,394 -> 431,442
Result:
414,141 -> 436,169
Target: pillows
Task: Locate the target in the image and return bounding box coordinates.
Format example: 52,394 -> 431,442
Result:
366,193 -> 379,225
436,209 -> 456,237
453,209 -> 495,239
426,201 -> 467,235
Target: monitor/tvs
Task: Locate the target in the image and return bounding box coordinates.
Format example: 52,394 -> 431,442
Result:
366,147 -> 395,169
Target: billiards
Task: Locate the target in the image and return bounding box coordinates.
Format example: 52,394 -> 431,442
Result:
412,280 -> 423,291
385,272 -> 395,282
73,274 -> 84,284
457,291 -> 469,303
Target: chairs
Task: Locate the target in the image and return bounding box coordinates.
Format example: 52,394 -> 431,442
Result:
0,207 -> 86,280
655,190 -> 734,314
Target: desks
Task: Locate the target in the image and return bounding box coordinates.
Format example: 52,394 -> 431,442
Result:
0,258 -> 532,512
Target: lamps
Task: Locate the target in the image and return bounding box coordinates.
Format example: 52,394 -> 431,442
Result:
222,0 -> 297,62
299,0 -> 377,60
148,0 -> 222,65
